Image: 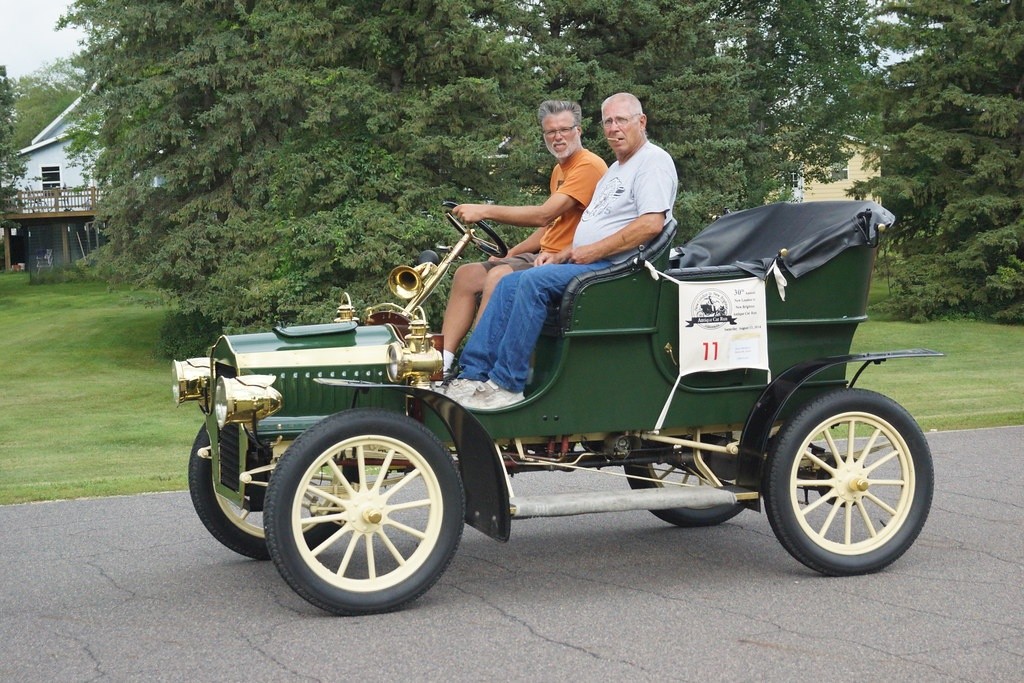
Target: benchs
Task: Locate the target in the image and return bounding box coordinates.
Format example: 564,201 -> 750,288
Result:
669,200 -> 896,330
541,219 -> 679,337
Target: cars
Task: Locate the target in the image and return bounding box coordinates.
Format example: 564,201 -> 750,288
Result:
171,198 -> 947,620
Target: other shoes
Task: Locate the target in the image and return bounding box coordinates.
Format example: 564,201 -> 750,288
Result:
431,368 -> 453,381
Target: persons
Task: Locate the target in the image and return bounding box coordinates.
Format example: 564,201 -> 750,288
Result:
412,100 -> 608,390
434,92 -> 679,409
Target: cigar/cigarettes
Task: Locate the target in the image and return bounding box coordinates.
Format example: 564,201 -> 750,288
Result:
606,138 -> 619,141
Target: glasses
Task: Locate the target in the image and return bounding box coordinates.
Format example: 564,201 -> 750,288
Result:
598,113 -> 641,129
543,123 -> 577,136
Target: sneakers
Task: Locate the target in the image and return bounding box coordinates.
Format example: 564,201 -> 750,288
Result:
439,378 -> 485,404
456,379 -> 525,411
430,377 -> 457,394
